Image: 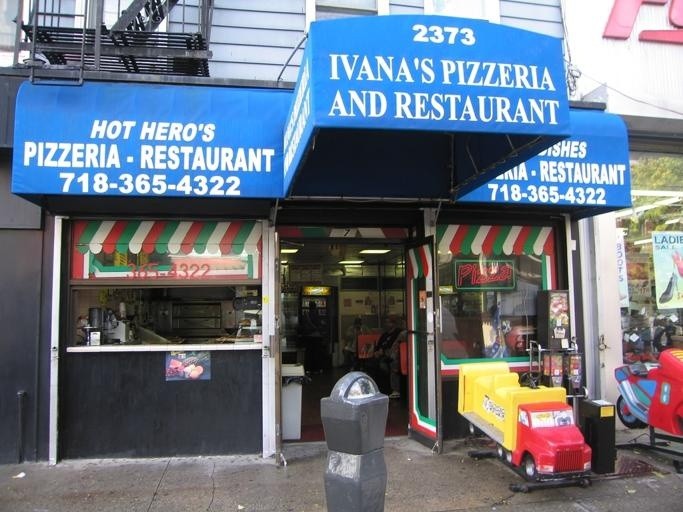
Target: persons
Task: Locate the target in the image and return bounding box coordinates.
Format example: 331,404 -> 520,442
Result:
343,317 -> 373,372
373,316 -> 403,395
441,305 -> 459,340
385,315 -> 408,399
302,301 -> 325,375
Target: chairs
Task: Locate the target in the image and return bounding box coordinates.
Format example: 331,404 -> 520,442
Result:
492,371 -> 520,399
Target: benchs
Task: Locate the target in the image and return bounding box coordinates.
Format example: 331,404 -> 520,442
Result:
355,327 -> 523,418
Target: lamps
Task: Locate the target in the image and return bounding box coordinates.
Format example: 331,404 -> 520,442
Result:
614,189 -> 683,246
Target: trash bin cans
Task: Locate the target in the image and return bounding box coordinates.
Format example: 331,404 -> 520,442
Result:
281,363 -> 304,440
